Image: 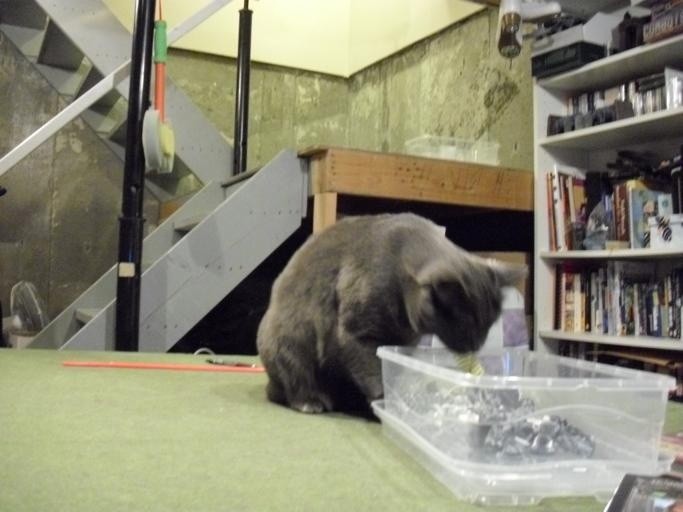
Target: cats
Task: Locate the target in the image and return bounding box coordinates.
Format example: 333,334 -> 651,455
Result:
254,209 -> 533,419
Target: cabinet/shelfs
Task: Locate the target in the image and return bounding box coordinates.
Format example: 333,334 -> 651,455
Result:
532,32 -> 683,401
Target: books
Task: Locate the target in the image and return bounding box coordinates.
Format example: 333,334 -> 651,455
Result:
613,427 -> 682,479
599,472 -> 682,512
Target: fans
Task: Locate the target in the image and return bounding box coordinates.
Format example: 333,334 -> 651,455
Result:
1,279 -> 50,348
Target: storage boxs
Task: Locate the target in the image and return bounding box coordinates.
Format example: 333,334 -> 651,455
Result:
530,22 -> 607,78
375,345 -> 677,507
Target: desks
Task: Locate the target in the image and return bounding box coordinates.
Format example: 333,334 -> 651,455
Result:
1,347 -> 682,511
